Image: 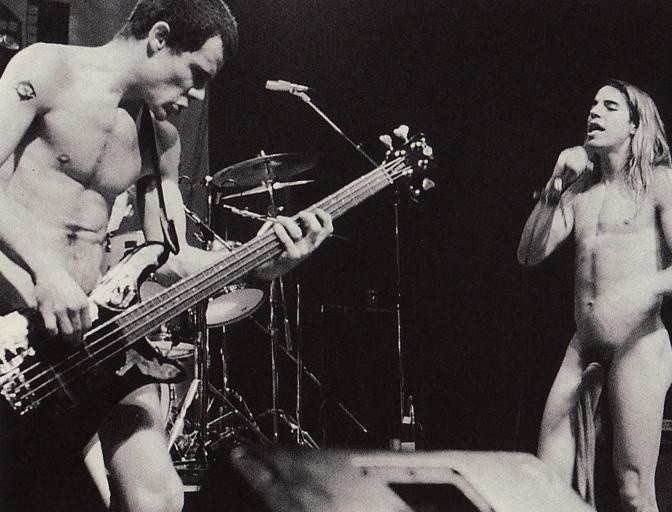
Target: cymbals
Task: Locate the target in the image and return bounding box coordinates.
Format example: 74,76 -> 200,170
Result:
207,148 -> 321,190
224,180 -> 314,200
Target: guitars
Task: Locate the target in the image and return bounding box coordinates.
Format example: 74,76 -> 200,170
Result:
0,119 -> 438,496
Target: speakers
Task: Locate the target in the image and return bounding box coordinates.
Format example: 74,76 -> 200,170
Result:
181,450 -> 598,512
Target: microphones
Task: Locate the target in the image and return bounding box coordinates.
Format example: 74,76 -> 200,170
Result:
264,79 -> 310,96
211,191 -> 223,230
548,143 -> 597,210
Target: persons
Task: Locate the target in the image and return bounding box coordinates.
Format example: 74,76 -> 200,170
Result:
512,74 -> 671,511
91,109 -> 187,511
0,0 -> 334,512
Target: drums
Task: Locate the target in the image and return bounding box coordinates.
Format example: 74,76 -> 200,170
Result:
185,238 -> 266,329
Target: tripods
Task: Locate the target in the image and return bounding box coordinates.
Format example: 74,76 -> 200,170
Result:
206,185 -> 320,454
184,325 -> 261,459
168,312 -> 272,456
